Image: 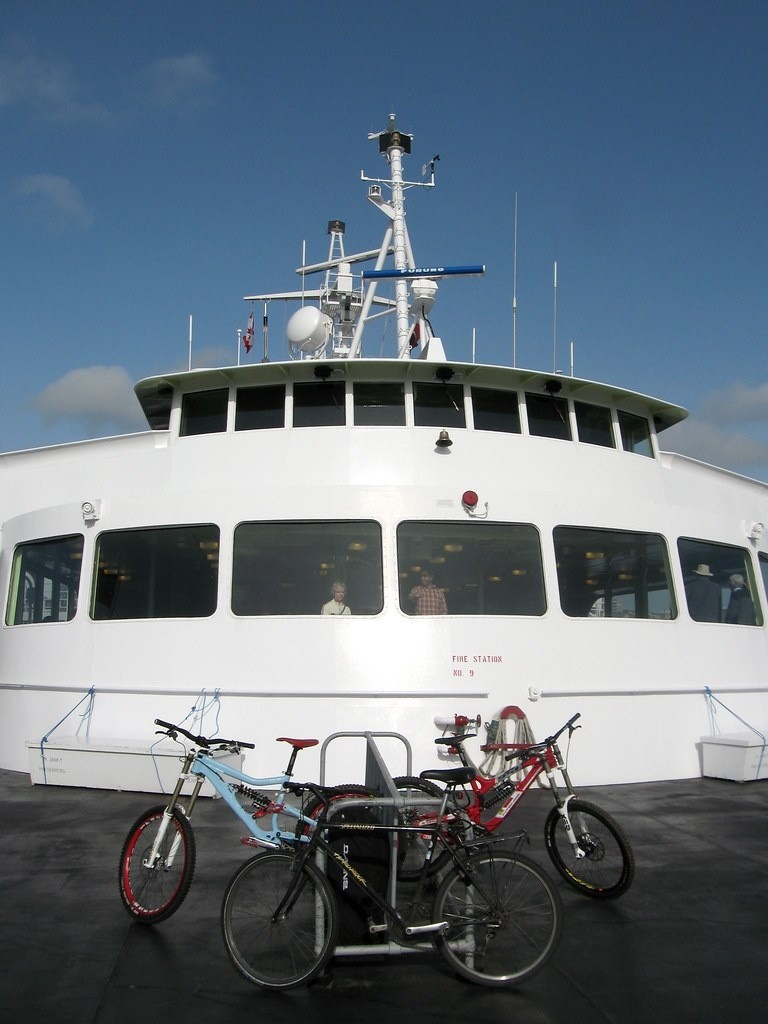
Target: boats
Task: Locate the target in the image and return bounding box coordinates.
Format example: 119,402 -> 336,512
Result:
0,105 -> 768,802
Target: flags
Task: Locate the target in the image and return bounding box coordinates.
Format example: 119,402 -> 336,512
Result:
243,315 -> 254,354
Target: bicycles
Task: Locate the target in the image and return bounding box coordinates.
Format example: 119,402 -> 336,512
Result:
394,712 -> 636,900
220,766 -> 565,988
118,718 -> 409,925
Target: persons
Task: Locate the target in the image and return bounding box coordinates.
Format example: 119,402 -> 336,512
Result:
685,564 -> 723,623
410,570 -> 449,615
727,575 -> 759,626
319,583 -> 352,616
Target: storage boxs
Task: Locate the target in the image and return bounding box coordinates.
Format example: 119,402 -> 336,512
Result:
26,742 -> 243,799
700,734 -> 768,781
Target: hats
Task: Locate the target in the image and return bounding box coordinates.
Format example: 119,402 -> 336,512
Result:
692,564 -> 713,576
